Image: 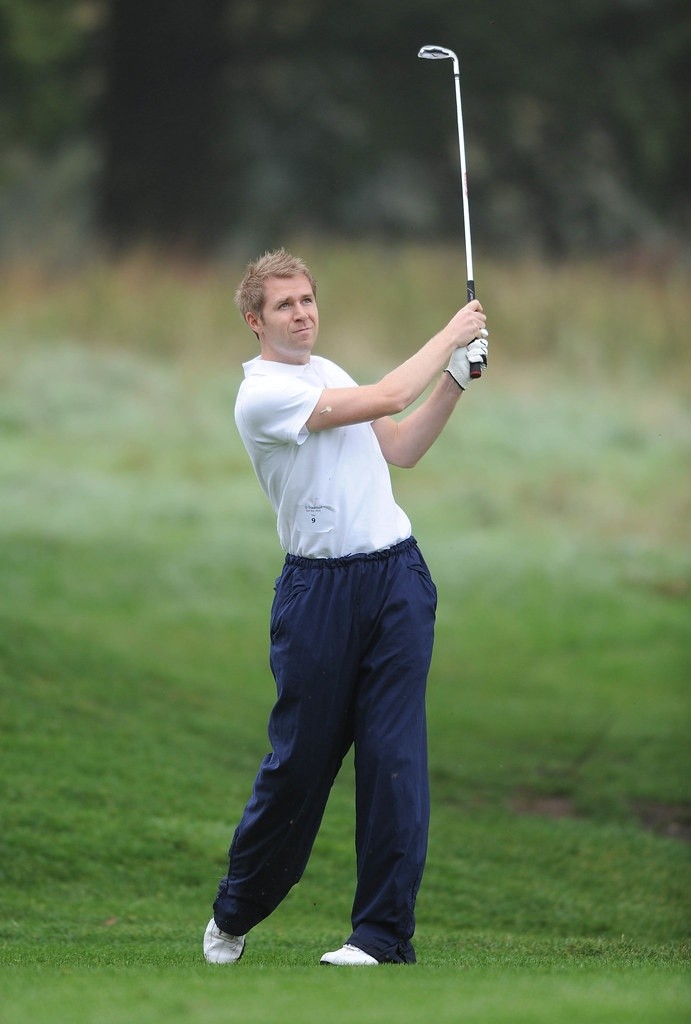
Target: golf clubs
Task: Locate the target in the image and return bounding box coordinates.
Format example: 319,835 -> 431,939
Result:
419,43 -> 487,380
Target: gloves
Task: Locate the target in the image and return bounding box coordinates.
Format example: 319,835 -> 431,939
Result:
444,328 -> 489,391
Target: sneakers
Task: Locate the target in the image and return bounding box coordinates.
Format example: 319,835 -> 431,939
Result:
202,918 -> 247,966
319,942 -> 381,967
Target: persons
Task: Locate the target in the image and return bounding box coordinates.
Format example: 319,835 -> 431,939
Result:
202,247 -> 489,967
